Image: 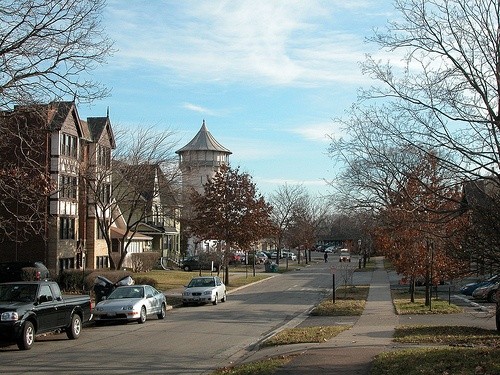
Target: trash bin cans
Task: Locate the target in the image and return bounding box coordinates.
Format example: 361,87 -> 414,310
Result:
93,275 -> 132,304
264,264 -> 278,273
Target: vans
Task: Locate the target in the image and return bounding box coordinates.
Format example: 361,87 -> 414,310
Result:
180,255 -> 219,272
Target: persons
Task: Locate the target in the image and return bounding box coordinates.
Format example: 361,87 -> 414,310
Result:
324,252 -> 328,263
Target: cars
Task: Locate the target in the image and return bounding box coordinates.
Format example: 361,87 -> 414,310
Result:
339,253 -> 351,263
0,261 -> 51,281
93,285 -> 166,324
182,275 -> 227,305
229,250 -> 296,264
311,243 -> 348,253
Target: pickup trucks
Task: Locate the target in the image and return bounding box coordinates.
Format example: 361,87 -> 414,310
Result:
0,281 -> 91,350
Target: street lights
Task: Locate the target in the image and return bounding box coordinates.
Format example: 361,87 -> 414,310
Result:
358,239 -> 361,269
